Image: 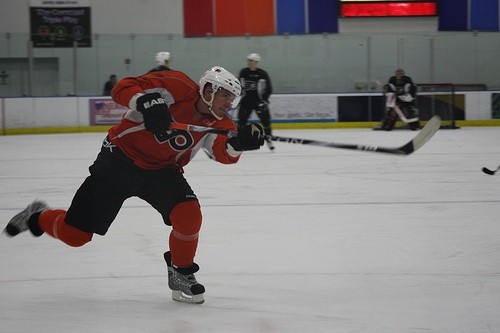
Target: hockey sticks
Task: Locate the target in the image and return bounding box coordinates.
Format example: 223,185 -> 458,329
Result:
170,116 -> 441,155
482,164 -> 500,177
376,79 -> 418,123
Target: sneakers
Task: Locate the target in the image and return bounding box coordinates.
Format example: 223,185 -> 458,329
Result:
3,200 -> 47,237
163,251 -> 205,304
266,139 -> 275,151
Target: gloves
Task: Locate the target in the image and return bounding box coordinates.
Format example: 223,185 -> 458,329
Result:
228,123 -> 264,151
257,100 -> 269,113
135,92 -> 173,132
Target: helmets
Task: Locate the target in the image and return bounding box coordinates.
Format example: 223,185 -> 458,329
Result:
247,53 -> 260,61
154,51 -> 170,65
198,66 -> 242,108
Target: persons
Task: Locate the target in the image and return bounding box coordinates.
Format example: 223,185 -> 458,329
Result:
102,74 -> 117,95
238,53 -> 275,153
148,51 -> 172,73
373,68 -> 422,130
4,65 -> 265,303
